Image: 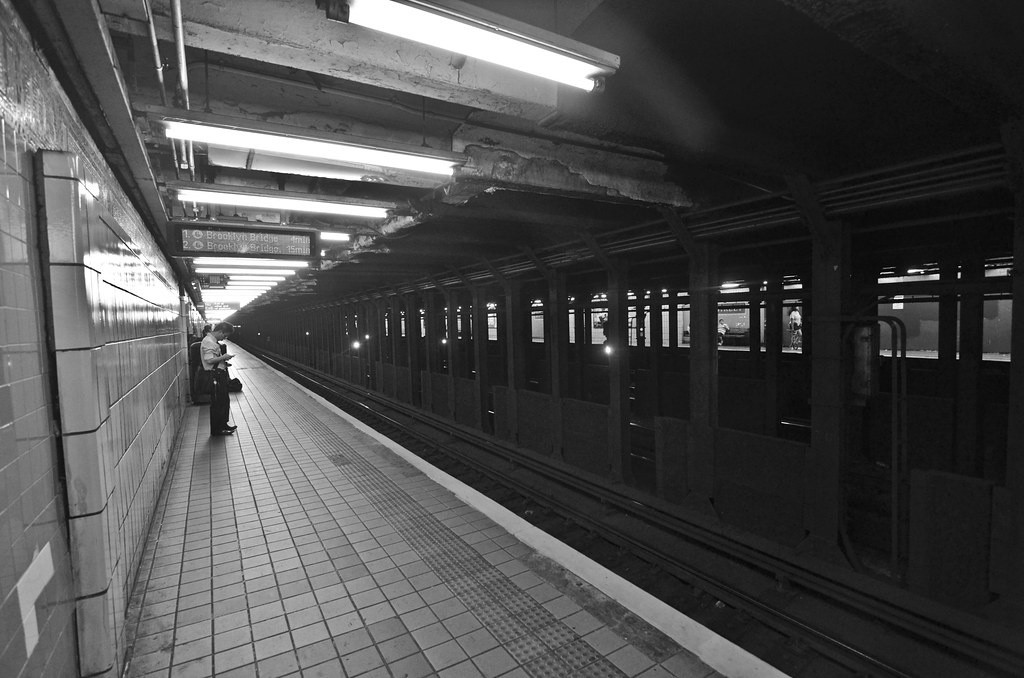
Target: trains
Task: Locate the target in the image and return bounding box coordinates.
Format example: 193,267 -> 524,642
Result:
226,133 -> 1024,678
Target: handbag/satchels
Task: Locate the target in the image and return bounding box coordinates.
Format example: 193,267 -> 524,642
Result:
194,368 -> 213,394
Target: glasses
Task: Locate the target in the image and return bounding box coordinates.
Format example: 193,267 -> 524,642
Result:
223,332 -> 228,339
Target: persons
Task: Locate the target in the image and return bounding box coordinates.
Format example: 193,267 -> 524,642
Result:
200,323 -> 238,435
788,305 -> 802,347
718,320 -> 730,345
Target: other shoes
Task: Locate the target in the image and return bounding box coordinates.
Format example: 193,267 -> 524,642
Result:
222,424 -> 237,430
220,429 -> 234,435
